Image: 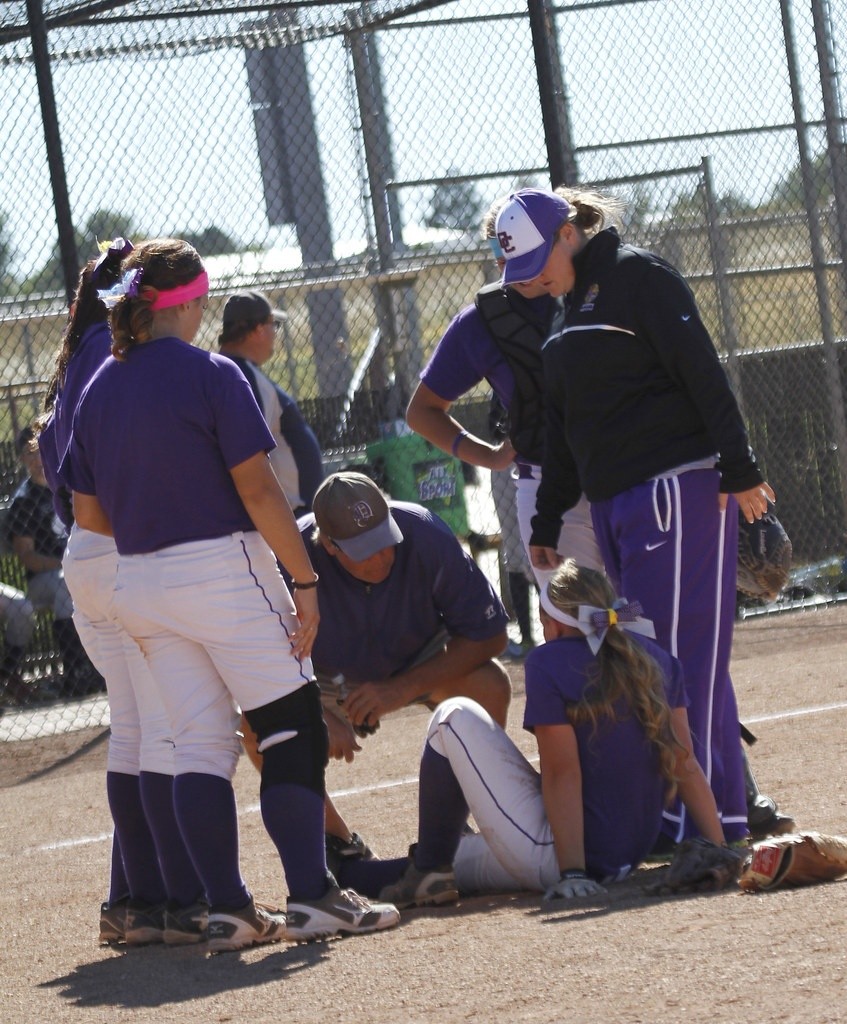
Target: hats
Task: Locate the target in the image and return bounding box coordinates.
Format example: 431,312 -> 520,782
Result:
15,426 -> 38,456
311,471 -> 404,563
222,289 -> 288,324
495,188 -> 570,288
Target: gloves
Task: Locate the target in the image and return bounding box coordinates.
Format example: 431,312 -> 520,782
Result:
543,868 -> 608,902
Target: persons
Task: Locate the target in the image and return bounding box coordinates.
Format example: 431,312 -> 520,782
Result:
0,186 -> 795,948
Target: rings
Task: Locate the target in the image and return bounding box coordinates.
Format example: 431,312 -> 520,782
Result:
309,626 -> 315,632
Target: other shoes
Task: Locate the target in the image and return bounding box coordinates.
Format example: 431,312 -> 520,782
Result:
757,811 -> 797,838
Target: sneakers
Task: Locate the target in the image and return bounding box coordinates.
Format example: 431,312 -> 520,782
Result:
124,897 -> 165,945
98,901 -> 127,944
378,860 -> 460,909
206,890 -> 287,950
162,895 -> 210,946
283,870 -> 403,945
351,831 -> 381,863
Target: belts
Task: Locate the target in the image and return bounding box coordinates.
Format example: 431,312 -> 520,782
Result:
514,462 -> 544,481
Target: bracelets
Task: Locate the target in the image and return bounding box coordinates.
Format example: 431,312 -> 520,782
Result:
453,430 -> 470,457
293,573 -> 321,589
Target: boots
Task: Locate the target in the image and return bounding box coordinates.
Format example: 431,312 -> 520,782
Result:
53,617 -> 105,697
0,639 -> 31,697
